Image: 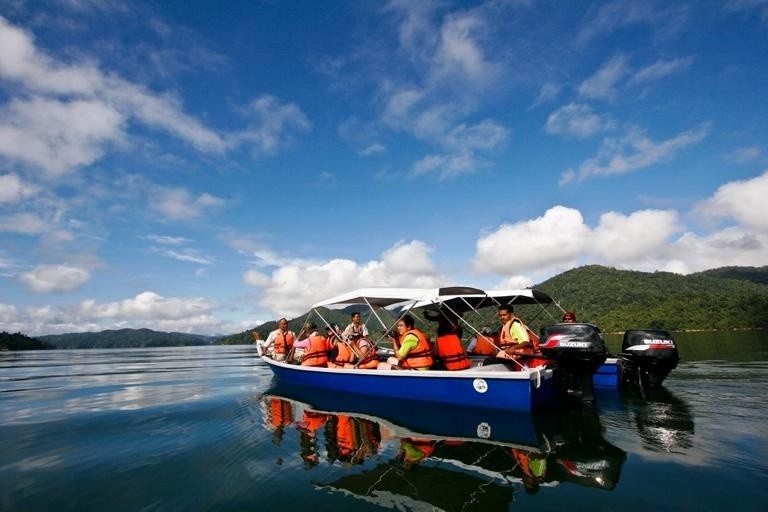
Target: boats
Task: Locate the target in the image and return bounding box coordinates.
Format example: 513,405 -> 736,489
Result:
257,387 -> 629,511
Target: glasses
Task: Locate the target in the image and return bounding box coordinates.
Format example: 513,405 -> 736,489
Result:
562,317 -> 573,320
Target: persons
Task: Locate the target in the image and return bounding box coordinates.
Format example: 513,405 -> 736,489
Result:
340,311 -> 369,340
396,434 -> 437,472
422,305 -> 464,336
291,320 -> 332,370
561,309 -> 580,324
258,318 -> 296,362
435,314 -> 473,369
506,446 -> 549,497
324,321 -> 343,349
384,314 -> 434,371
494,302 -> 542,371
256,395 -> 299,451
294,405 -> 323,469
324,414 -> 385,471
465,325 -> 500,356
345,326 -> 380,370
